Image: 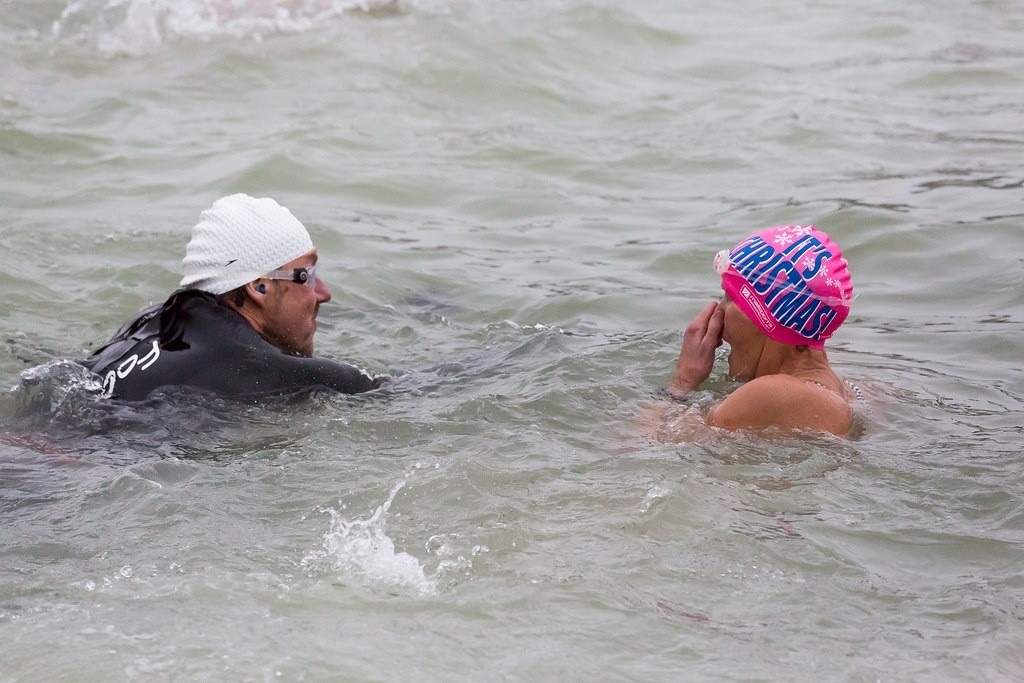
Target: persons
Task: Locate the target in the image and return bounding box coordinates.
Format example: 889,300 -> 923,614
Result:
672,224 -> 855,436
91,194 -> 378,403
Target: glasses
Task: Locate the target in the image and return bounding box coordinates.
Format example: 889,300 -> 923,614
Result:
712,250 -> 860,314
184,264 -> 318,289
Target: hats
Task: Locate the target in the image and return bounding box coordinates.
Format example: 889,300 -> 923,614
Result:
713,224 -> 854,350
178,193 -> 313,295
